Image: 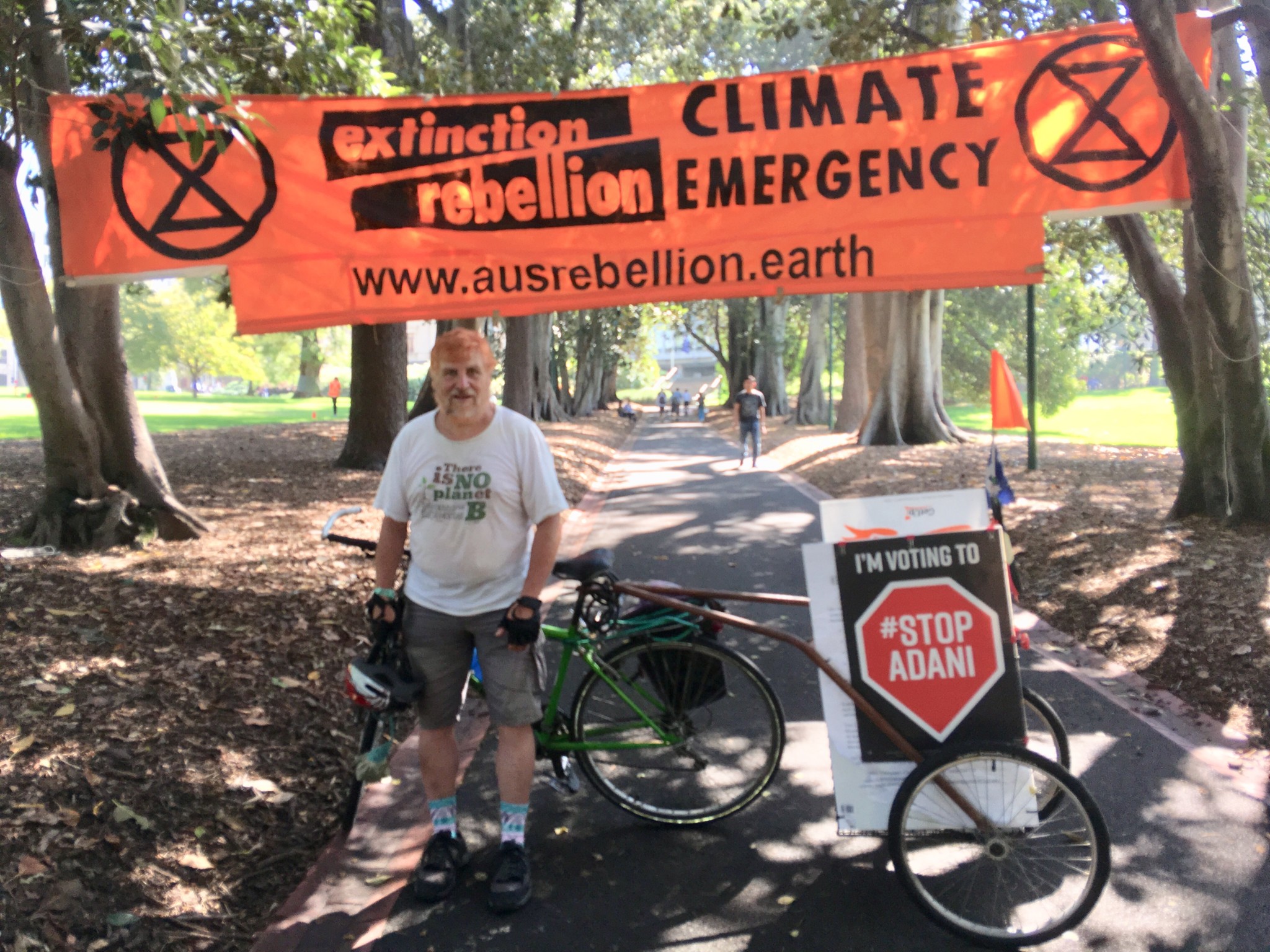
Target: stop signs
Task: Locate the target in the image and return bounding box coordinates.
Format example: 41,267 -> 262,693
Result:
853,577 -> 1008,746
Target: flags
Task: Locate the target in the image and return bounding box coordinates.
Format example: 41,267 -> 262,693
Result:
985,446 -> 1016,511
990,350 -> 1031,430
1002,530 -> 1022,604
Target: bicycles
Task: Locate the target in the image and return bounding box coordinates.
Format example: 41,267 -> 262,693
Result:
318,506 -> 788,836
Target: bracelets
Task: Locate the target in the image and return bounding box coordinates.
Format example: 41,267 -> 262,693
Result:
373,588 -> 395,598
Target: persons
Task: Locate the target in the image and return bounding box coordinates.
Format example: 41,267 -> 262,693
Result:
328,377 -> 340,415
682,390 -> 691,416
674,389 -> 682,414
656,389 -> 667,417
733,375 -> 766,467
670,393 -> 677,413
617,400 -> 636,422
697,394 -> 705,420
363,328 -> 570,914
623,400 -> 632,413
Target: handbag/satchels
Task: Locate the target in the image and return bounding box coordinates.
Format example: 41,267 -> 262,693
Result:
630,599 -> 729,711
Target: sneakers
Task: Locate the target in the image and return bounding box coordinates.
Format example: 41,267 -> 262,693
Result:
490,838 -> 529,909
418,830 -> 466,900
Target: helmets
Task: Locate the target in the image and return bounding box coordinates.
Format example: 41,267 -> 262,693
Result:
345,655 -> 426,713
